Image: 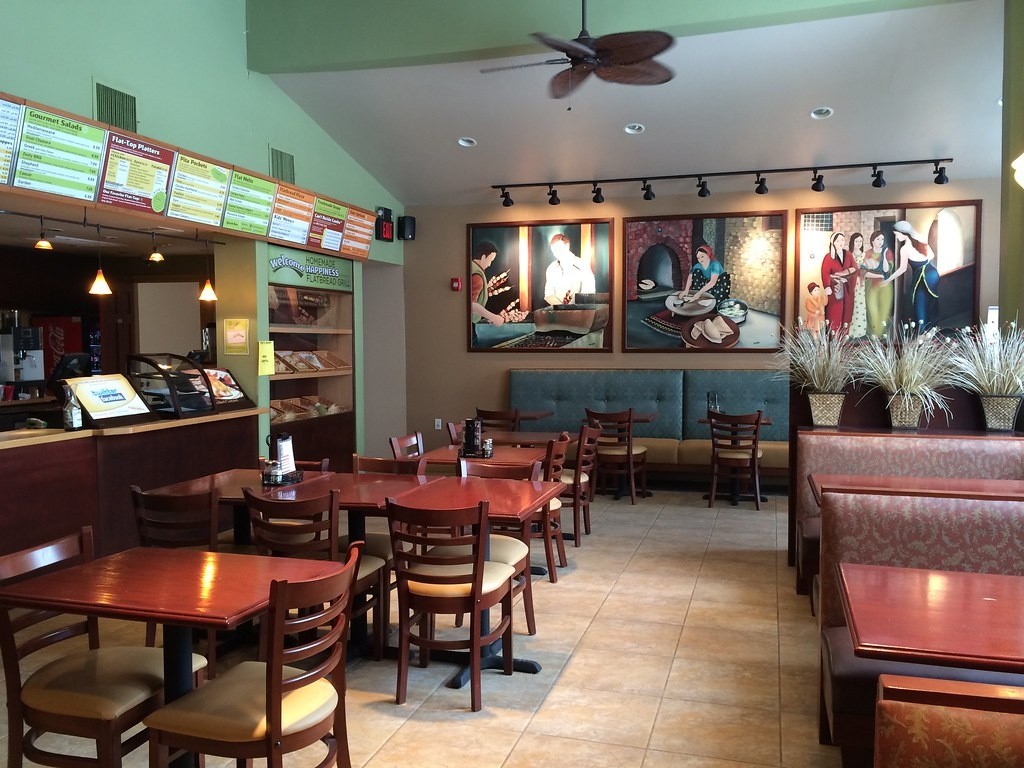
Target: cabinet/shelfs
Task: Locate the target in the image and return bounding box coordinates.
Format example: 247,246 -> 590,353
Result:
464,218 -> 614,352
268,286 -> 358,470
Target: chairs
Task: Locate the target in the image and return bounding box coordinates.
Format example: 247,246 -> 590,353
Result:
706,410 -> 764,510
0,405 -> 637,768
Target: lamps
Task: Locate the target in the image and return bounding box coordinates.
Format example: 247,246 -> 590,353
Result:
35,216 -> 53,250
497,187 -> 514,207
151,234 -> 165,263
89,226 -> 112,297
641,180 -> 656,201
870,166 -> 886,188
591,183 -> 604,204
199,240 -> 218,302
753,172 -> 768,193
695,177 -> 710,199
934,161 -> 948,184
546,184 -> 561,205
810,169 -> 826,192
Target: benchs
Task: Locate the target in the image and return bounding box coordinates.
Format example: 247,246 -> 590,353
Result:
872,672 -> 1024,766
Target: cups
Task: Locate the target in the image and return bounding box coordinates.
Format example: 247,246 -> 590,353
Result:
0,385 -> 4,401
3,385 -> 15,401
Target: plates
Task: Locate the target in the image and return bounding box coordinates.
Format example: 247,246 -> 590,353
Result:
203,387 -> 244,400
189,375 -> 236,393
180,368 -> 230,379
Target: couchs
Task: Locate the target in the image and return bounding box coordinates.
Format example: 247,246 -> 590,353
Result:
508,366 -> 790,478
817,488 -> 1024,745
792,430 -> 1024,596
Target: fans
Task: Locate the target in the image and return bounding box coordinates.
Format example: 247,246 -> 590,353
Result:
480,0 -> 675,98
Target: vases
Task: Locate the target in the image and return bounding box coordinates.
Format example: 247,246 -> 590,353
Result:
806,391 -> 848,429
977,393 -> 1023,433
886,394 -> 924,430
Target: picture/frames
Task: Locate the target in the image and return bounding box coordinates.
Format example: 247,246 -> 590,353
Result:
619,210 -> 788,354
793,199 -> 982,344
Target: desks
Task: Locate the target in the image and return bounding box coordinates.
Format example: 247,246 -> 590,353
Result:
582,412 -> 657,500
480,431 -> 580,446
260,472 -> 446,640
412,445 -> 548,478
836,561 -> 1024,670
385,476 -> 568,634
518,409 -> 554,431
808,471 -> 1024,508
0,546 -> 345,768
143,467 -> 337,557
698,417 -> 772,506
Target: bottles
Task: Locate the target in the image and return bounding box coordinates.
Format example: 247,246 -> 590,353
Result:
270,461 -> 282,485
488,438 -> 493,454
62,384 -> 83,431
35,387 -> 39,398
263,460 -> 272,484
482,440 -> 490,457
88,328 -> 101,345
88,348 -> 101,370
